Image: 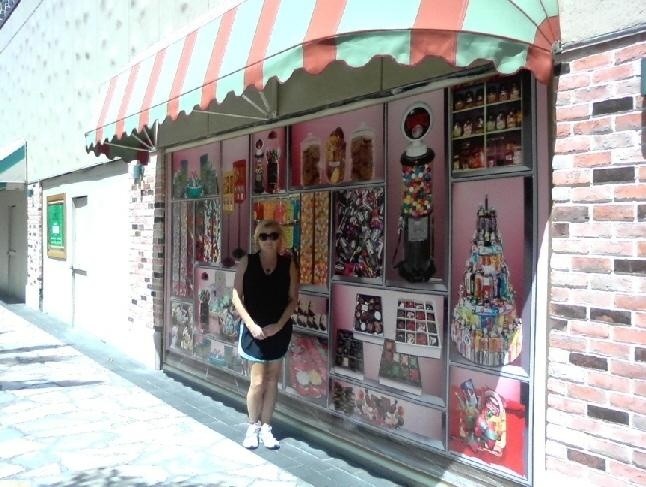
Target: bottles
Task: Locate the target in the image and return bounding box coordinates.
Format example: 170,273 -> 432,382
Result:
452,83 -> 522,137
299,126 -> 377,186
253,132 -> 279,193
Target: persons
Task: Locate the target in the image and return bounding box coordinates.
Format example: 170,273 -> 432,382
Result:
230,219 -> 301,450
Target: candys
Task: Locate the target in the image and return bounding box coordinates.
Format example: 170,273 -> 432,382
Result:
401,160 -> 433,218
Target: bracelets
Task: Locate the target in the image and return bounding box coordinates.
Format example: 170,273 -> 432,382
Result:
279,318 -> 282,329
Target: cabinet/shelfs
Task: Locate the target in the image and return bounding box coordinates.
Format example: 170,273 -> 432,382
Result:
161,66 -> 536,487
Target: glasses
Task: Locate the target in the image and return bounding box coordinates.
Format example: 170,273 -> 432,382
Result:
258,232 -> 280,240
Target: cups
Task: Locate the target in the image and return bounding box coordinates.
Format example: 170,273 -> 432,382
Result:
186,183 -> 203,199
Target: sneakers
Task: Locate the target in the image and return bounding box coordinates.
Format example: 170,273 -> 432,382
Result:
260,423 -> 280,448
242,423 -> 259,448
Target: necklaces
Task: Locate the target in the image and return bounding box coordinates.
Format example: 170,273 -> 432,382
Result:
265,267 -> 272,274
